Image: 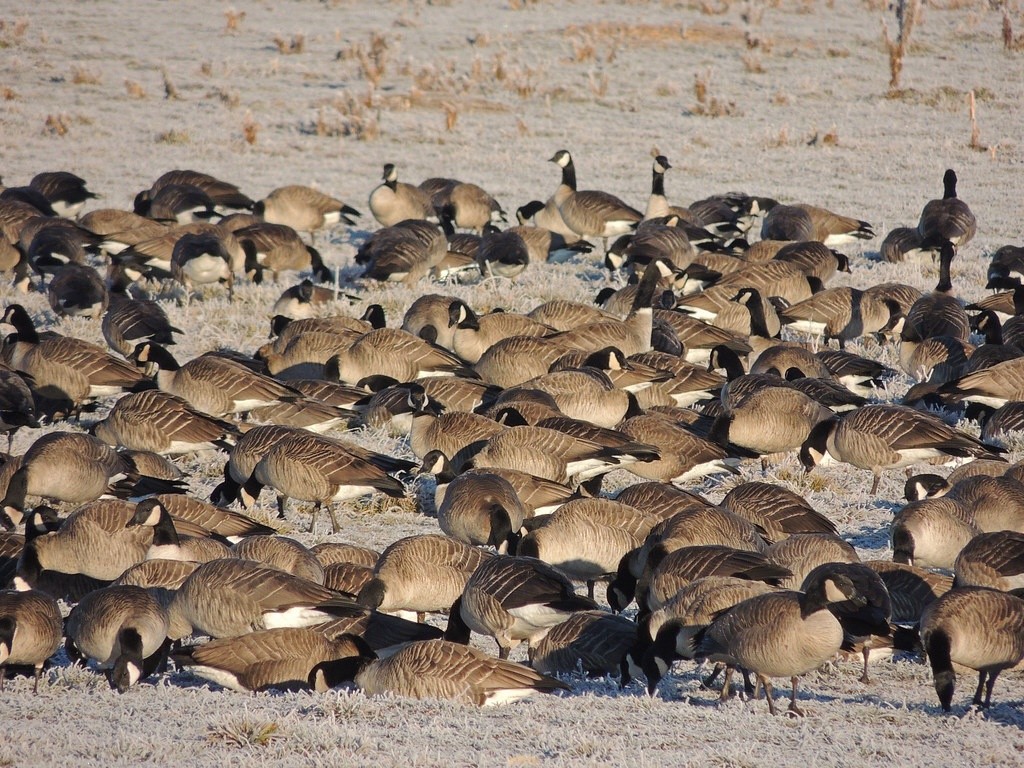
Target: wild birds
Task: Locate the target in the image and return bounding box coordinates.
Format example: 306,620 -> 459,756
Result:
0,150 -> 1024,720
